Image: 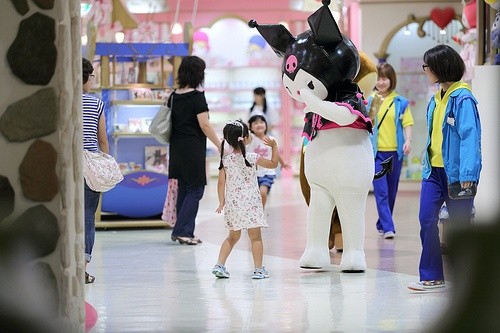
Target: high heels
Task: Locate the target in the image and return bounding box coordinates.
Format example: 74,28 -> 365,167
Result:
171,234 -> 202,245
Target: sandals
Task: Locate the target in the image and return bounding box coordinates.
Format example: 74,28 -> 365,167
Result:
252,265 -> 269,278
212,263 -> 230,278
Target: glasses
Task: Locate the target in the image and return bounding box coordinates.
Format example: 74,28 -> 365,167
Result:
422,64 -> 431,72
89,74 -> 95,80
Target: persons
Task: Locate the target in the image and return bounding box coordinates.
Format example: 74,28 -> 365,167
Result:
407,45 -> 481,292
82,58 -> 109,284
167,55 -> 226,245
243,115 -> 289,229
211,119 -> 277,278
365,63 -> 414,239
248,87 -> 274,136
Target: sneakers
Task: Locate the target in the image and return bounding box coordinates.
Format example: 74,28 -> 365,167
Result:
408,278 -> 445,290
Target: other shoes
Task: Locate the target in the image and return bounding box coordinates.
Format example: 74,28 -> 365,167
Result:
84,272 -> 95,283
378,229 -> 385,235
384,231 -> 395,239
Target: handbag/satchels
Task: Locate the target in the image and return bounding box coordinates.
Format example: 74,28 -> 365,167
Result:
83,149 -> 124,192
148,90 -> 175,145
161,178 -> 179,225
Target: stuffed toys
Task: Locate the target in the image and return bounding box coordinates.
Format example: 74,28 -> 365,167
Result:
452,0 -> 478,88
248,0 -> 375,272
299,53 -> 384,250
485,0 -> 500,65
